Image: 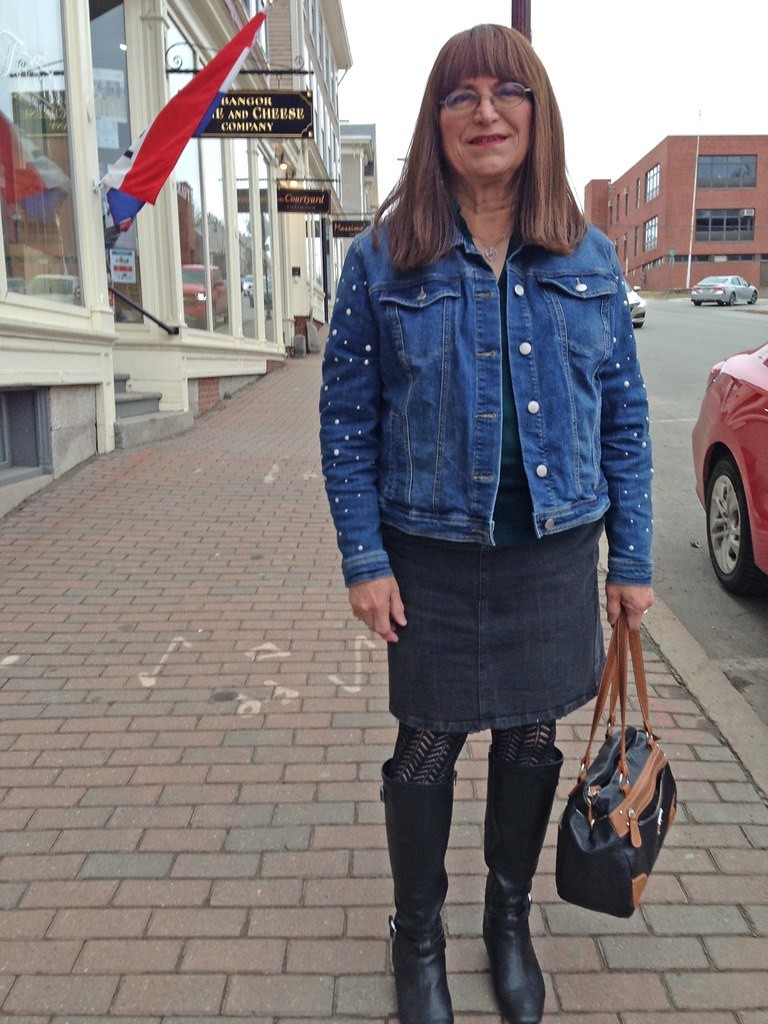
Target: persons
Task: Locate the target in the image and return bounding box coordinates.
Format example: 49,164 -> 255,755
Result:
319,23 -> 652,1024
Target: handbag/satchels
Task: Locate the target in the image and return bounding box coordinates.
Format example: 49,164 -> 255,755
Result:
555,612 -> 679,918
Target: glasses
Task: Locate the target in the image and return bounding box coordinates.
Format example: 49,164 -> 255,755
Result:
438,82 -> 532,115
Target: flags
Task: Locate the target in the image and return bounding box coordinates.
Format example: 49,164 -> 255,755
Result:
0,112 -> 70,224
101,12 -> 267,233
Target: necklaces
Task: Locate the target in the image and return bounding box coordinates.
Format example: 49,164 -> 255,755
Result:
476,235 -> 503,262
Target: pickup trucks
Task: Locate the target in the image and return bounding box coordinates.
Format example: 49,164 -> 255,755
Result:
182,265 -> 228,330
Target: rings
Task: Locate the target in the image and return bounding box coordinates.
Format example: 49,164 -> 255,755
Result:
643,608 -> 648,614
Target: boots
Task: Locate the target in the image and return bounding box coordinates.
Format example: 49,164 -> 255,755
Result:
483,743 -> 566,1024
378,759 -> 458,1024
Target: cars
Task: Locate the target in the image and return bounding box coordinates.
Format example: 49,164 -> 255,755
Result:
242,275 -> 272,307
689,275 -> 759,306
692,341 -> 768,595
8,278 -> 26,294
27,275 -> 80,305
624,280 -> 646,328
